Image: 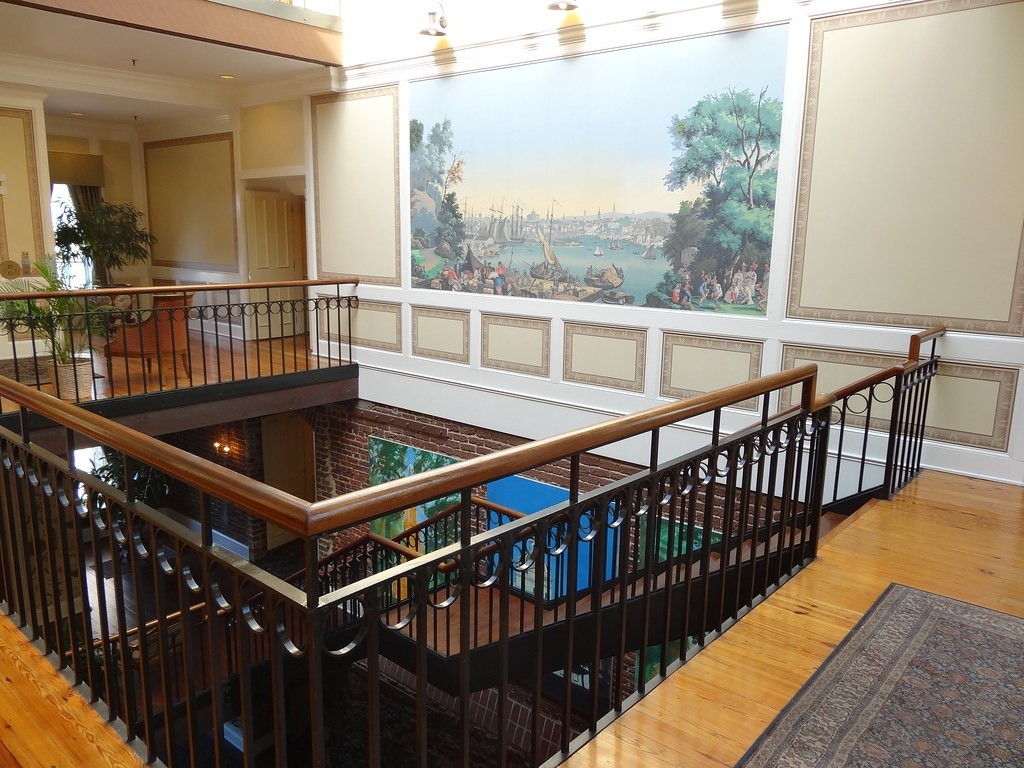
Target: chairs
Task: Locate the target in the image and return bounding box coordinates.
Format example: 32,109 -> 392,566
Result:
104,294 -> 194,379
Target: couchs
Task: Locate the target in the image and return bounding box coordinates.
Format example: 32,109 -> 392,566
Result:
0,276 -> 65,359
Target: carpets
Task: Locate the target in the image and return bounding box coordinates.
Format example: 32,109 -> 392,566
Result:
0,354 -> 105,385
90,560 -> 132,579
734,582 -> 1024,768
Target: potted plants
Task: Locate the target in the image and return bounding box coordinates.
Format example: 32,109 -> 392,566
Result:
53,194 -> 157,321
0,253 -> 122,400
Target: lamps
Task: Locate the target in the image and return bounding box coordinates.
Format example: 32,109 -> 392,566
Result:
419,3 -> 447,38
